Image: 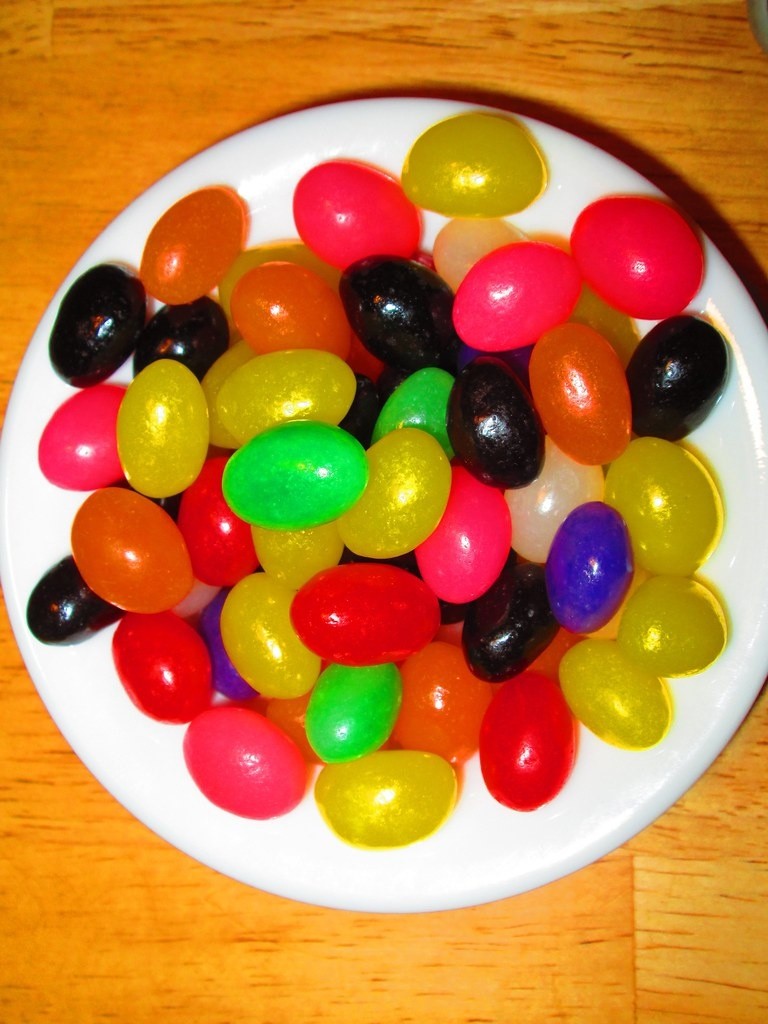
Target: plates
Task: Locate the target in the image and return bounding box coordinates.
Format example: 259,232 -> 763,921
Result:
0,97 -> 768,914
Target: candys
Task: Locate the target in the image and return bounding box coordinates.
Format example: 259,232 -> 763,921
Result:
16,113 -> 736,846
